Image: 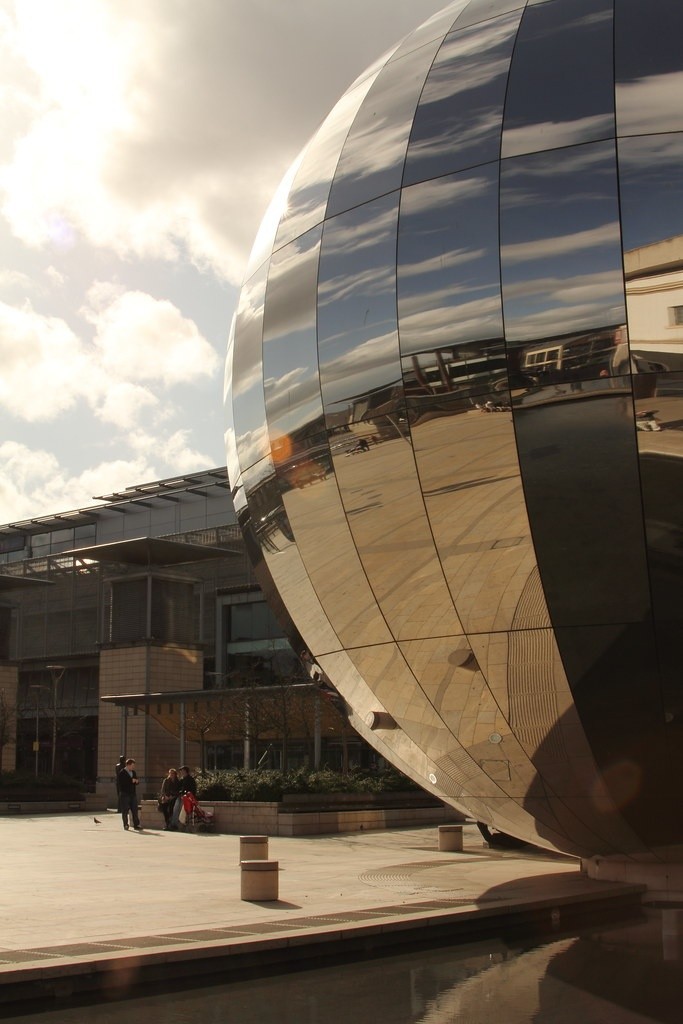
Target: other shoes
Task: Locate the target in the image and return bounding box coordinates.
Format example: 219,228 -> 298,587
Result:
124,825 -> 129,830
133,825 -> 141,830
163,826 -> 168,830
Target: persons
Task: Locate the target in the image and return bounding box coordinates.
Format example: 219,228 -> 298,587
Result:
161,768 -> 180,830
357,439 -> 369,452
164,766 -> 196,831
635,409 -> 662,432
116,758 -> 142,830
475,401 -> 511,412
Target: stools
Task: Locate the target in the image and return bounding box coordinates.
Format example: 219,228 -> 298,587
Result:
241,860 -> 278,901
438,825 -> 464,852
239,836 -> 269,862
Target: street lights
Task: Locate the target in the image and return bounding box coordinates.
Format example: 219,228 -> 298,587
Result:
30,684 -> 45,778
46,665 -> 67,778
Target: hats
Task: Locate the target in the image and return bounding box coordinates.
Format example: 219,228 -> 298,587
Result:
178,766 -> 189,770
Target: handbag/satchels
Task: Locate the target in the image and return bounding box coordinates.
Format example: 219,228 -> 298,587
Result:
157,794 -> 167,813
179,804 -> 187,824
